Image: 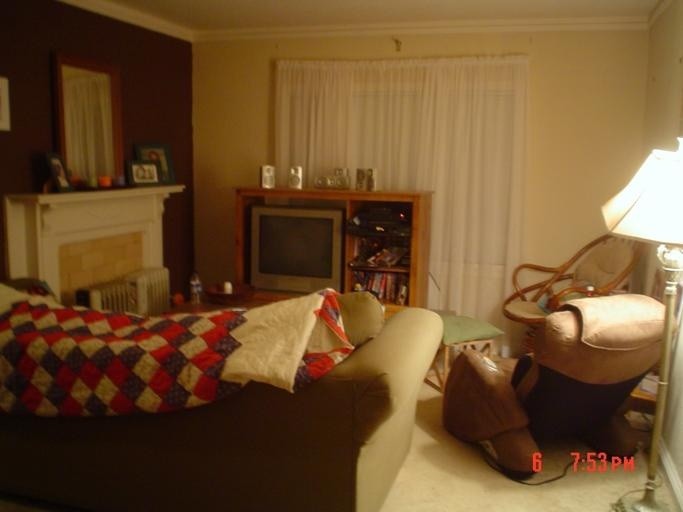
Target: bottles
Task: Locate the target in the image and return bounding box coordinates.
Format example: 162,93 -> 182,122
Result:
188,274 -> 203,305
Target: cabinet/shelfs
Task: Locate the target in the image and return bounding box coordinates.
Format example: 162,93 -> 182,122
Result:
233,185 -> 435,319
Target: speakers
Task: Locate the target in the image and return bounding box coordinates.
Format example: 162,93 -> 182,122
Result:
314,175 -> 351,190
260,165 -> 276,189
335,167 -> 349,176
287,166 -> 303,190
355,168 -> 373,191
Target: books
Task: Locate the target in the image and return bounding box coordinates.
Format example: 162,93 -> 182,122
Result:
351,241 -> 410,306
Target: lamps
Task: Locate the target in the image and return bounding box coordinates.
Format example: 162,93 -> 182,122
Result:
600,135 -> 683,511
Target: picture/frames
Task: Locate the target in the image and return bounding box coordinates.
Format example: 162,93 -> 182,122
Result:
126,143 -> 174,188
41,153 -> 72,193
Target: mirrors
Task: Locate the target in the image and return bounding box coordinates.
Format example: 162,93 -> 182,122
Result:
50,52 -> 127,190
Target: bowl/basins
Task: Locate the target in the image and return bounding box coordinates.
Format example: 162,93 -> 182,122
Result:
204,284 -> 253,306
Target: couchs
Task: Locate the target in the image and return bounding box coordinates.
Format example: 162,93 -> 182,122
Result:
0,282 -> 442,512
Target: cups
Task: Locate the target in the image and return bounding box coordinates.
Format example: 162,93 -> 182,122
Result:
89,173 -> 126,187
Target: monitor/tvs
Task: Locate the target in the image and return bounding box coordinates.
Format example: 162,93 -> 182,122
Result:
251,206 -> 343,294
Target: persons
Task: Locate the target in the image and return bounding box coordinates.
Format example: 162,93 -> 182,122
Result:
135,166 -> 145,179
148,150 -> 167,179
